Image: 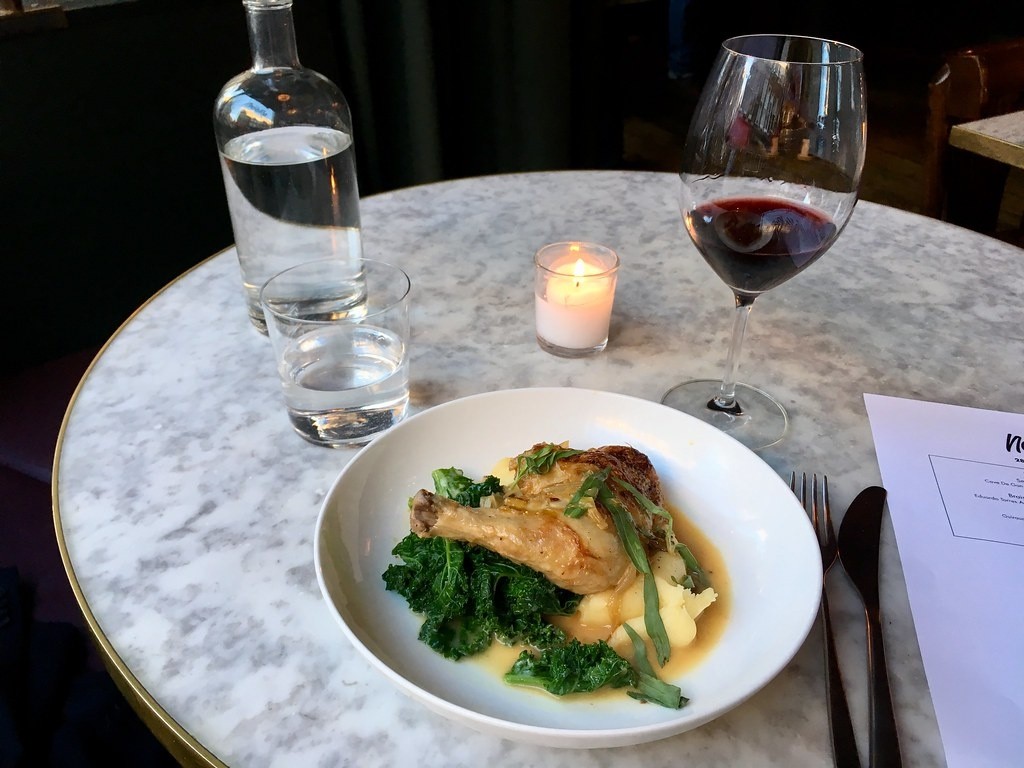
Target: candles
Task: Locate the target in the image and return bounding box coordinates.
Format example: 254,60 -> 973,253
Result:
533,241 -> 620,359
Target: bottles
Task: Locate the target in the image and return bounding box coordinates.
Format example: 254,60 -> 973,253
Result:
214,1 -> 367,337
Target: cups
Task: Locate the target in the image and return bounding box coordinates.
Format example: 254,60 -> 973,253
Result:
534,241 -> 622,358
260,256 -> 414,448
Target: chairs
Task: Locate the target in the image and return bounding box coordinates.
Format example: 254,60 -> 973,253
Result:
922,38 -> 1024,246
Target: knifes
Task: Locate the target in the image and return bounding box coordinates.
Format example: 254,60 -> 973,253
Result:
839,486 -> 903,768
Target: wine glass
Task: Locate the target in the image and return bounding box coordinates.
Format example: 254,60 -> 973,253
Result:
675,34 -> 868,450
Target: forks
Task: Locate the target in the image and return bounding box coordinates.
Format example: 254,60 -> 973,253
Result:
789,470 -> 862,768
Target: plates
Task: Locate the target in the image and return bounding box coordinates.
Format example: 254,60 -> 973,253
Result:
311,384 -> 825,750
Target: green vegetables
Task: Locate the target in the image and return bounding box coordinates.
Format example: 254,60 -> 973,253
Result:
380,444 -> 707,710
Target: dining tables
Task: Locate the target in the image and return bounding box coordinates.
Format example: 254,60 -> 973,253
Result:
50,170 -> 1024,768
